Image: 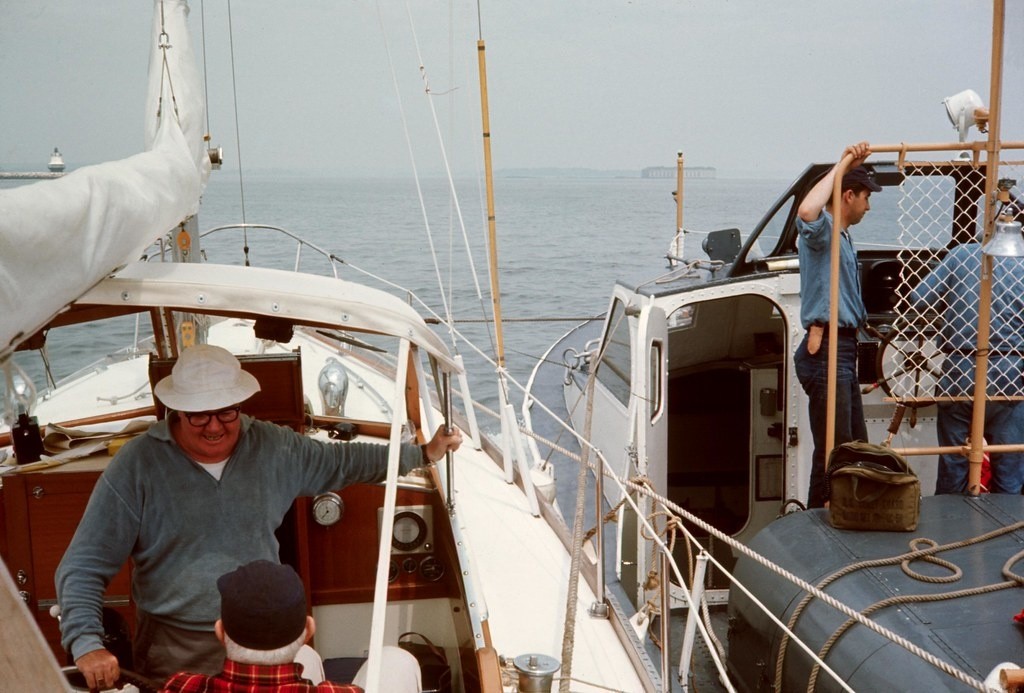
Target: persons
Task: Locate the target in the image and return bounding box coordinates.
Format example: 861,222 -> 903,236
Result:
157,559 -> 421,693
792,141 -> 883,510
55,344 -> 463,693
911,202 -> 1024,495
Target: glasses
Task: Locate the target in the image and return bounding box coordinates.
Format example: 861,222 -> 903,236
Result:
183,406 -> 241,427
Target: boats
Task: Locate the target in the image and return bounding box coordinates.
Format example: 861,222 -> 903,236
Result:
523,0 -> 1024,693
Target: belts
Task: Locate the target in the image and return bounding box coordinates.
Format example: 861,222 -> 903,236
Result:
807,326 -> 859,339
946,348 -> 1024,356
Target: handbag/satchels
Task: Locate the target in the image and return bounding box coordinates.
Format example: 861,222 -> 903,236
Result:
826,438 -> 920,531
398,632 -> 452,693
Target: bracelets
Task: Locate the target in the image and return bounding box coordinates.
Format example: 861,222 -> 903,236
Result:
421,444 -> 431,465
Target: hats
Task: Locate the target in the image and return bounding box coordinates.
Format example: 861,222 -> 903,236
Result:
217,559 -> 307,651
842,166 -> 882,192
154,345 -> 261,412
